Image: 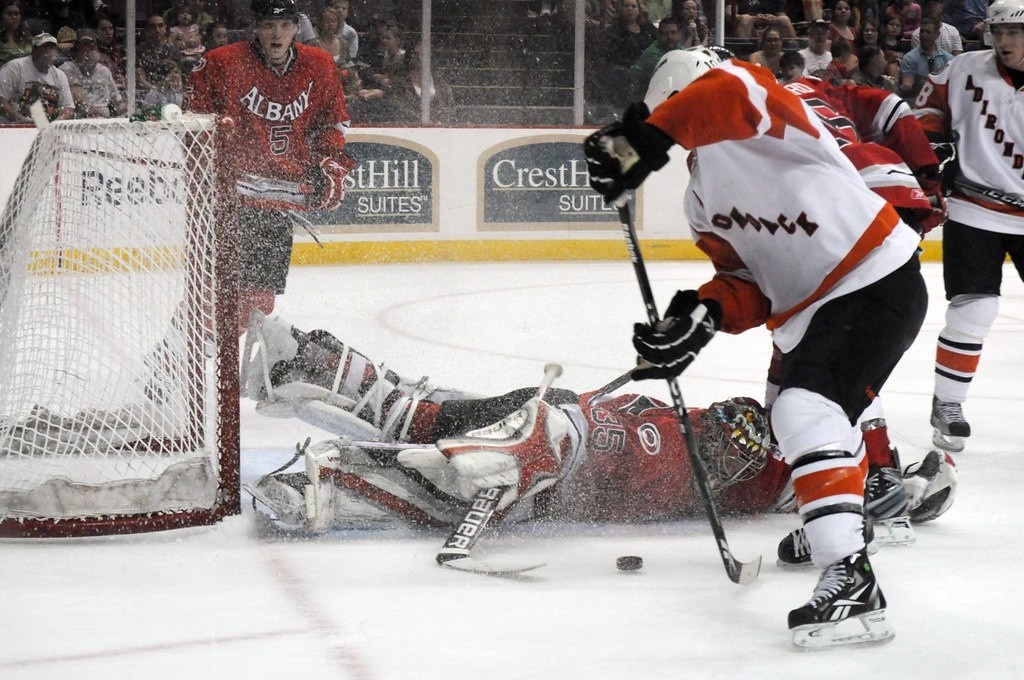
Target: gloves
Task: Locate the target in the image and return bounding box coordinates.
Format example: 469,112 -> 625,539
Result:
926,133 -> 958,197
584,103 -> 675,204
915,176 -> 949,233
398,399 -> 584,508
311,147 -> 356,211
630,287 -> 723,381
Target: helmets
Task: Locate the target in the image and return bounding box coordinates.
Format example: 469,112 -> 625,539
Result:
982,0 -> 1024,24
251,0 -> 299,19
643,44 -> 722,115
701,397 -> 771,491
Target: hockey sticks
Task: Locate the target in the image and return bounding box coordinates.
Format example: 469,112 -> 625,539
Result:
432,359 -> 566,578
26,79 -> 186,171
598,132 -> 764,587
952,175 -> 1024,211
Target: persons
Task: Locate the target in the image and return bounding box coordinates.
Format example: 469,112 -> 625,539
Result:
0,0 -> 457,126
249,313 -> 772,539
913,0 -> 1024,438
584,46 -> 947,630
562,0 -> 994,109
143,0 -> 351,404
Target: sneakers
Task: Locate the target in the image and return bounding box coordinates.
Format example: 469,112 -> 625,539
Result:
777,482 -> 878,570
865,447 -> 915,546
930,395 -> 970,453
145,343 -> 176,406
789,549 -> 895,648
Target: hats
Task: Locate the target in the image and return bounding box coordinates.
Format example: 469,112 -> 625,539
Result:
811,18 -> 831,31
32,33 -> 58,47
74,28 -> 99,48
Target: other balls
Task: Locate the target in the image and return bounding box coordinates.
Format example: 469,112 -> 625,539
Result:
615,555 -> 644,572
